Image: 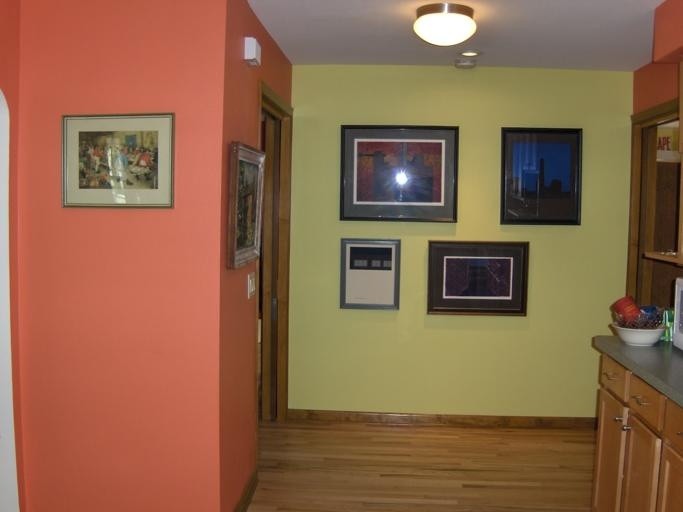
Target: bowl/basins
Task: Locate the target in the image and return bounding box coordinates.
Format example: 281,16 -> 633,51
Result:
613,311 -> 663,329
609,322 -> 666,347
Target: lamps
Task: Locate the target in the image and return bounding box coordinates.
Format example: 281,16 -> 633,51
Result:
413,4 -> 476,50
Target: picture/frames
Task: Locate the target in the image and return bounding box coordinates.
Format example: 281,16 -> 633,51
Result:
339,237 -> 402,310
58,112 -> 175,209
500,125 -> 583,225
426,239 -> 529,318
227,140 -> 267,270
340,124 -> 459,222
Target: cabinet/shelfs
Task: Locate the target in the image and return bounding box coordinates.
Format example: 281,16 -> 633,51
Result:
591,354 -> 682,511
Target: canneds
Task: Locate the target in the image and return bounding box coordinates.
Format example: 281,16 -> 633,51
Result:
611,295 -> 641,323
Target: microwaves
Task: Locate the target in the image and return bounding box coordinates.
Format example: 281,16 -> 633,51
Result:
672,276 -> 683,352
